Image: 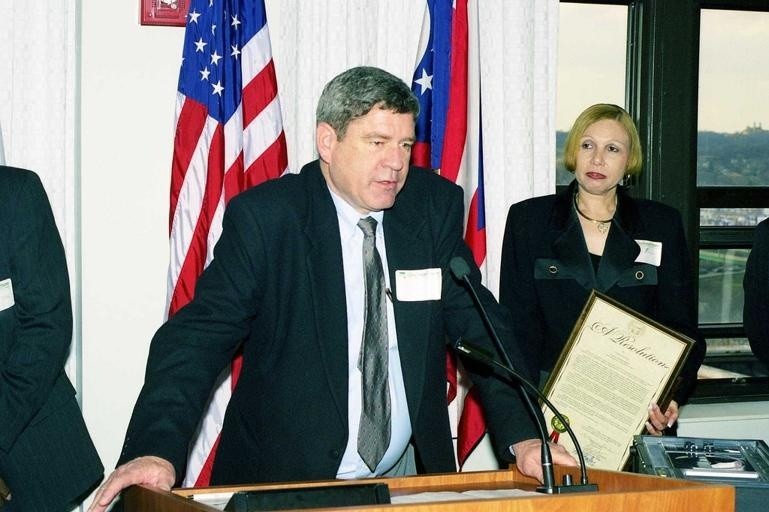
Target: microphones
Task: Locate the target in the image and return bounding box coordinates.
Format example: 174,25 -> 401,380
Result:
450,256 -> 555,488
453,338 -> 588,485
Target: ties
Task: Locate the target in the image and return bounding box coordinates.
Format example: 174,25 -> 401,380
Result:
353,217 -> 392,471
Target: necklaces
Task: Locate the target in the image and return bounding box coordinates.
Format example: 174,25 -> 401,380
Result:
574,193 -> 613,234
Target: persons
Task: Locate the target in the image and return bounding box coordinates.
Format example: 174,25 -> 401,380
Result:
88,65 -> 579,512
741,216 -> 769,371
498,102 -> 708,473
0,164 -> 106,512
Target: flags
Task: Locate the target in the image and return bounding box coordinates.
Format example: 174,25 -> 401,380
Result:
164,0 -> 291,489
409,0 -> 488,469
